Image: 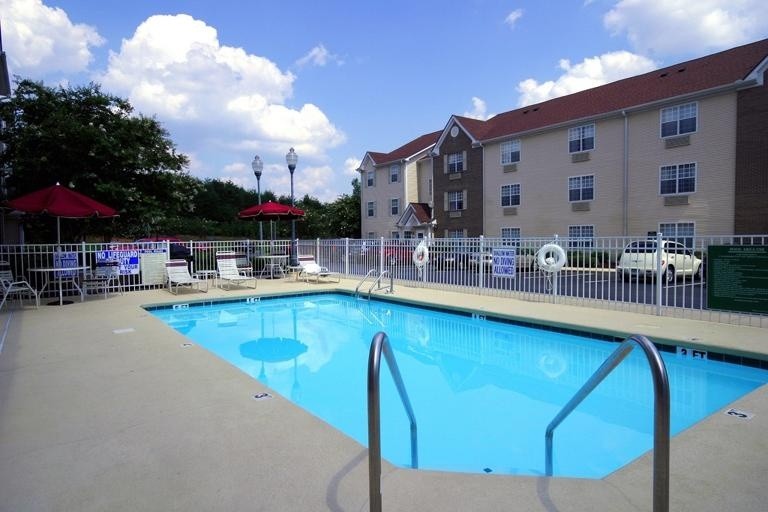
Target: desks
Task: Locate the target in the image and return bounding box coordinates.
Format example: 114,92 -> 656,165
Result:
27,266 -> 92,306
255,254 -> 288,279
196,270 -> 217,289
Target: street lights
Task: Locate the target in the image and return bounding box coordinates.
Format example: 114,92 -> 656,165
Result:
252,153 -> 265,240
284,147 -> 300,266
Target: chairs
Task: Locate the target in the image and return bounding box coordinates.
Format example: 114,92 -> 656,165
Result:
215,250 -> 257,290
168,296 -> 331,328
297,255 -> 340,285
80,259 -> 124,300
164,260 -> 209,295
0,260 -> 37,313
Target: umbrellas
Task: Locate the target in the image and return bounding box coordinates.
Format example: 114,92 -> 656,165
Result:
238,199 -> 304,279
7,181 -> 123,306
239,312 -> 308,363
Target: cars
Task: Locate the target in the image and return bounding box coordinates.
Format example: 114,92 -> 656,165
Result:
332,238 -> 542,275
617,239 -> 705,282
79,236 -> 261,280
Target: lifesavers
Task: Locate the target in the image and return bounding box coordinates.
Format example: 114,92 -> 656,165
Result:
537,243 -> 566,273
413,246 -> 428,266
